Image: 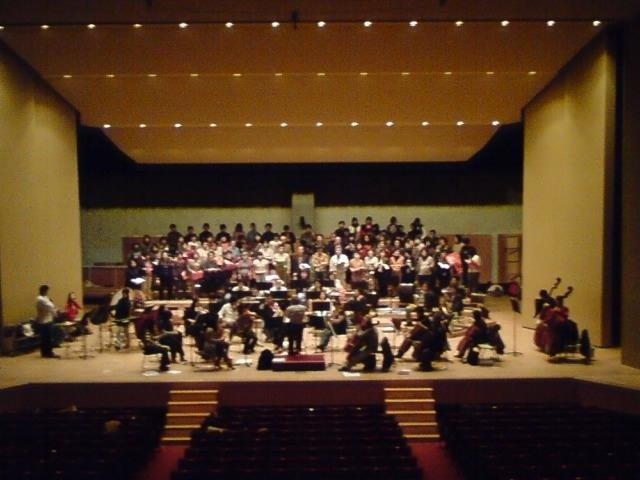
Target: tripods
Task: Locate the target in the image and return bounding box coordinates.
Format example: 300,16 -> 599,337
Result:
384,281 -> 399,316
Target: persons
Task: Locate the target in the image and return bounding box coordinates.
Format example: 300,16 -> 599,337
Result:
111,217 -> 506,373
36,284 -> 63,358
510,275 -> 576,362
65,291 -> 82,342
